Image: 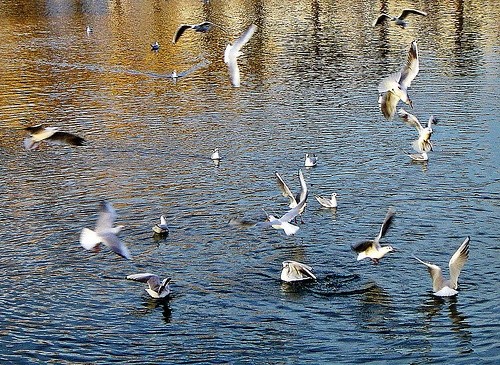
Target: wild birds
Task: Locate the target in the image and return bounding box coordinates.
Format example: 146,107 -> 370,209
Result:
315,192 -> 338,208
304,154 -> 316,167
378,40 -> 419,119
398,108 -> 439,160
411,235 -> 471,297
127,273 -> 172,297
352,207 -> 396,264
170,71 -> 178,78
173,21 -> 227,43
274,169 -> 309,224
80,199 -> 134,260
211,148 -> 221,160
281,260 -> 317,282
86,26 -> 91,31
154,215 -> 169,232
254,198 -> 308,235
313,153 -> 318,161
372,8 -> 427,29
223,23 -> 256,86
23,124 -> 87,150
150,41 -> 160,51
